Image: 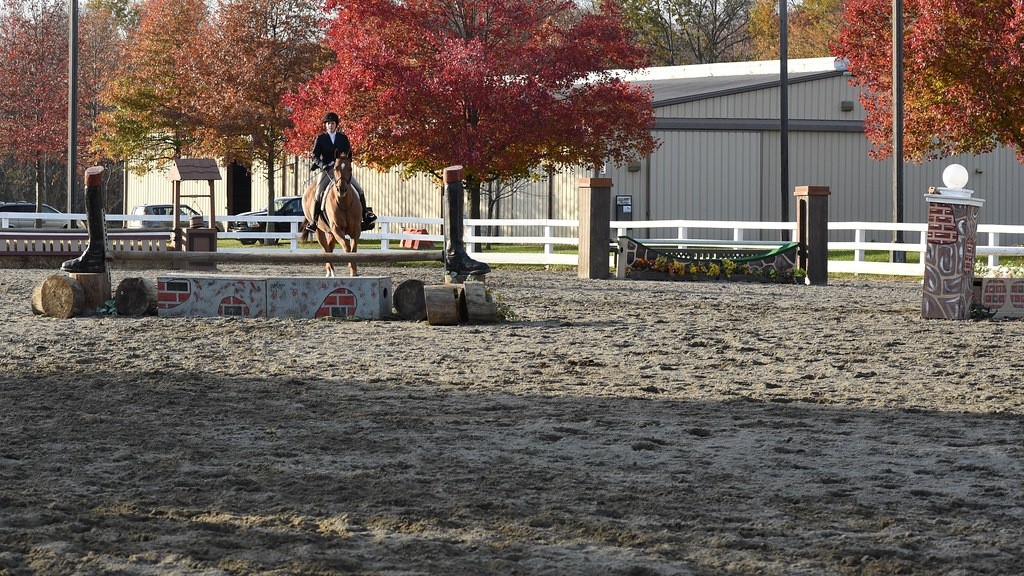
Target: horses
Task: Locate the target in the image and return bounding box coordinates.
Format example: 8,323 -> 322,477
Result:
302,149 -> 364,277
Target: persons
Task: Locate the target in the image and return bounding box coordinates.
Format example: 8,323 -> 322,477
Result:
306,112 -> 376,233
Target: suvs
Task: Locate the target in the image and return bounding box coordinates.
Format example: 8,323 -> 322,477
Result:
126,204 -> 225,240
0,200 -> 88,230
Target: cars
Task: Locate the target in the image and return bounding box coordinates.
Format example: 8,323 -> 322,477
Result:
230,195 -> 304,245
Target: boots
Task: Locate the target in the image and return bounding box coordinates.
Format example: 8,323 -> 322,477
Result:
360,193 -> 377,224
305,199 -> 321,232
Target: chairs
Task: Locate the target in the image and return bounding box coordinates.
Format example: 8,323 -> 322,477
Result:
160,209 -> 166,215
289,202 -> 299,211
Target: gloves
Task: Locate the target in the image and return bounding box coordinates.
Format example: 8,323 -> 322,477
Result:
322,165 -> 328,171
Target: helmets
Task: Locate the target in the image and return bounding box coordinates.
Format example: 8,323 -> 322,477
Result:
323,113 -> 338,122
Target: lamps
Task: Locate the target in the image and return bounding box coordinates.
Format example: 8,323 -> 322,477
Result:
976,168 -> 984,175
942,164 -> 968,189
